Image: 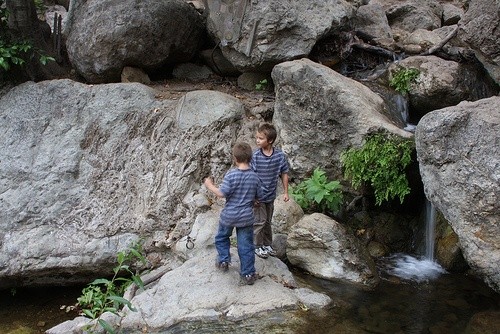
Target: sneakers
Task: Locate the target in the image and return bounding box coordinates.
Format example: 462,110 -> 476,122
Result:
262,244 -> 277,256
254,245 -> 268,259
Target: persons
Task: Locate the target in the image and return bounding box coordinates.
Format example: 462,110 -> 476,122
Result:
204,143 -> 265,285
248,123 -> 290,259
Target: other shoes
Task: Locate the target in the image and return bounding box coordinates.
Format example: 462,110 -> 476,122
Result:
215,261 -> 228,272
241,273 -> 255,285
